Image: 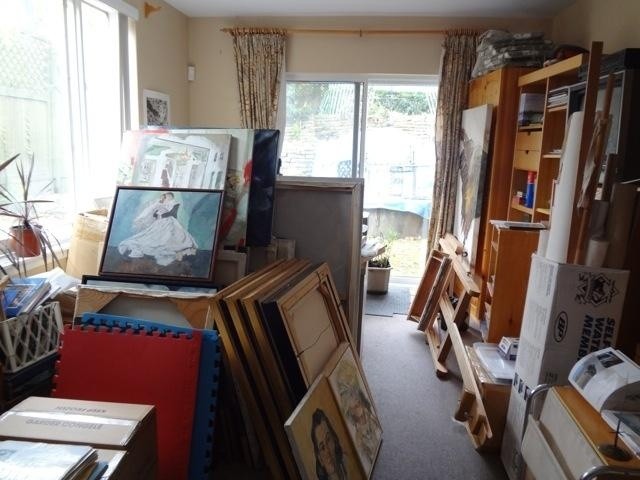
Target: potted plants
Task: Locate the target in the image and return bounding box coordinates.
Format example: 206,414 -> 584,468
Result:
366,228 -> 400,295
0,150 -> 67,304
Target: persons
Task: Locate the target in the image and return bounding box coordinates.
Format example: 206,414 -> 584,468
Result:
311,408 -> 352,479
0,274 -> 52,318
118,192 -> 197,267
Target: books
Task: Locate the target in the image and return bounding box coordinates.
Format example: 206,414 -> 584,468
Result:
547,148 -> 562,155
546,91 -> 569,109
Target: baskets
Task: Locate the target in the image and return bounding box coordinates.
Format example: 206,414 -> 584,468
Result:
0,300 -> 64,373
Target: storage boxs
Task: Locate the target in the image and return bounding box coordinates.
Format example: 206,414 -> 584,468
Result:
0,396 -> 157,480
86,448 -> 137,480
66,209 -> 110,282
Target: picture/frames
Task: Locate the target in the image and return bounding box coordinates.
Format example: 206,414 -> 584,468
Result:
208,258 -> 387,480
80,274 -> 226,297
98,185 -> 226,285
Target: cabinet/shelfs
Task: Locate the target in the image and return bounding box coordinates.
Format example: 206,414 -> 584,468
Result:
520,383 -> 640,480
467,67 -> 538,321
505,52 -> 587,223
482,223 -> 542,345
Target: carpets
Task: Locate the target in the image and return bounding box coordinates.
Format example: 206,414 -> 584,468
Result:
366,286 -> 412,317
